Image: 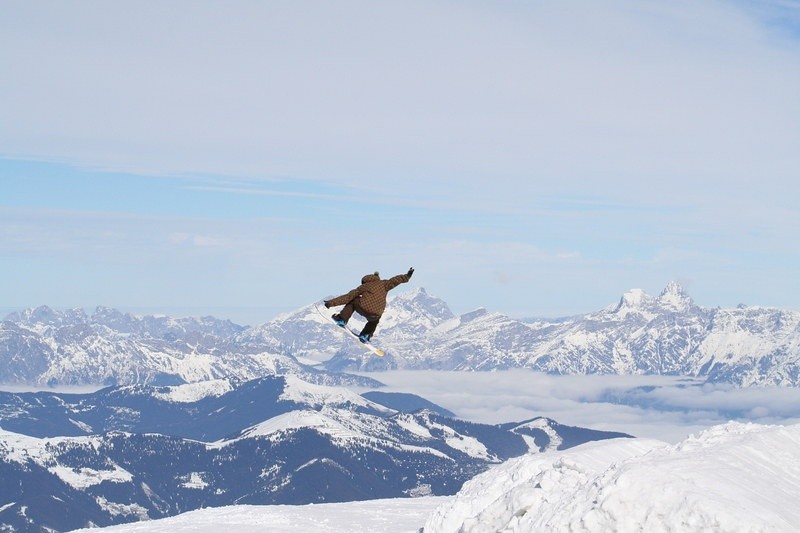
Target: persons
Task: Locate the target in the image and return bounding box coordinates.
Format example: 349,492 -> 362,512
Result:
323,266 -> 415,343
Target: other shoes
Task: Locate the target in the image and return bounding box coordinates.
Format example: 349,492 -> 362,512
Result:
332,314 -> 346,327
359,331 -> 370,343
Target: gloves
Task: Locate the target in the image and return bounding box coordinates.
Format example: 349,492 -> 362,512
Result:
407,267 -> 414,277
324,301 -> 330,309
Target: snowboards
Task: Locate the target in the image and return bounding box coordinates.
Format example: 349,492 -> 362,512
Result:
313,303 -> 385,358
